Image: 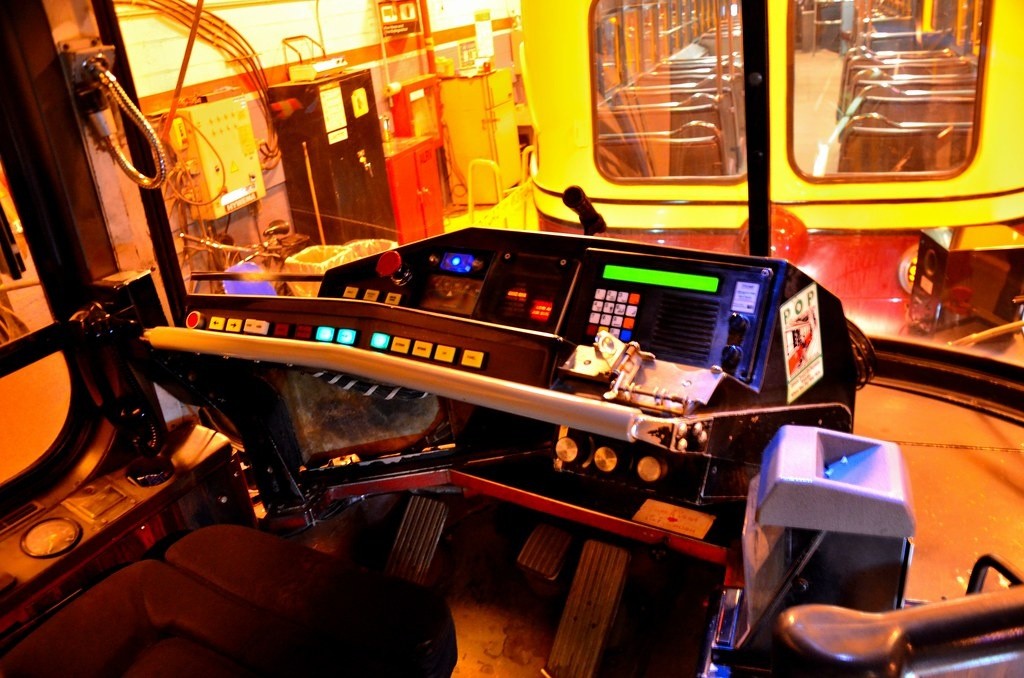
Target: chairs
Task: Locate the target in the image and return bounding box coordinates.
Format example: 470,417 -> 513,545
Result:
0,523 -> 458,678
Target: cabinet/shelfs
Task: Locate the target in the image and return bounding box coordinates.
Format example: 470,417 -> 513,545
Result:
386,138 -> 445,247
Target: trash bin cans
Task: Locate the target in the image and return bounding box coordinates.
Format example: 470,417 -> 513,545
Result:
908,223 -> 1024,334
284,244 -> 351,297
345,239 -> 398,262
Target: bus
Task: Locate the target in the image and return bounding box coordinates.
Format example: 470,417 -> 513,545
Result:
518,2 -> 1023,364
